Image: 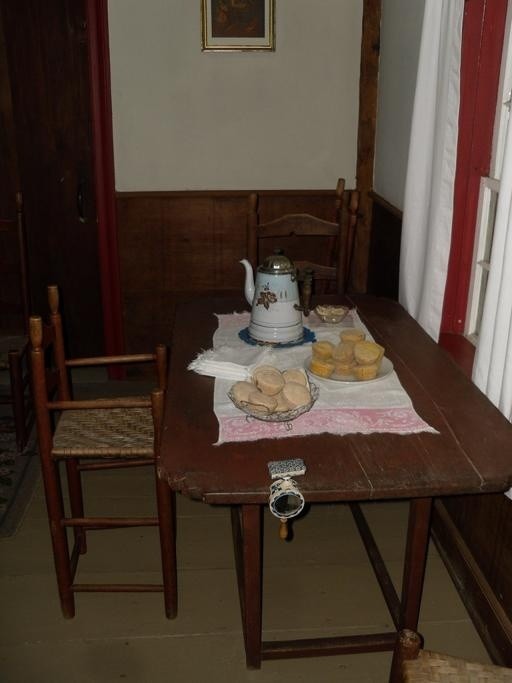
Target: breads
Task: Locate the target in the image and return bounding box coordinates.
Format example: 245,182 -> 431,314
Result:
231,366 -> 312,413
308,331 -> 385,381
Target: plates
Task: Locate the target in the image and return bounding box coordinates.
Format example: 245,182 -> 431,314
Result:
304,354 -> 395,384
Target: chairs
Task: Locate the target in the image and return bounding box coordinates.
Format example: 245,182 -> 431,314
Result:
244,176 -> 360,293
27,282 -> 181,619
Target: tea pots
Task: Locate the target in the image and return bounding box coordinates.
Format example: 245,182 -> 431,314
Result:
237,247 -> 315,344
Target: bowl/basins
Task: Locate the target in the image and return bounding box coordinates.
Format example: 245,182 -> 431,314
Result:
317,302 -> 348,324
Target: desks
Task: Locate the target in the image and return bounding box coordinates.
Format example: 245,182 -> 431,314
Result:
153,289 -> 511,672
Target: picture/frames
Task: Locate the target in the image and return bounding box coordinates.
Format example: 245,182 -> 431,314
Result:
199,0 -> 277,55
